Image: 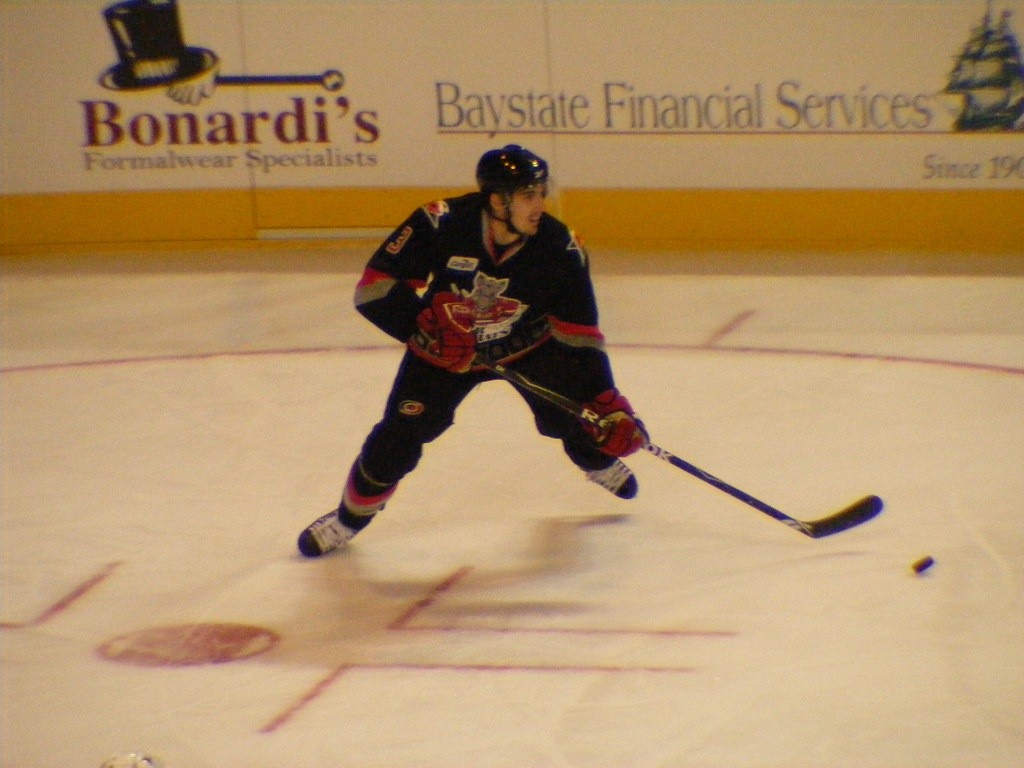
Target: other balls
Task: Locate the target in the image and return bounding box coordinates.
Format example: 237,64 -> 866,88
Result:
913,555 -> 934,573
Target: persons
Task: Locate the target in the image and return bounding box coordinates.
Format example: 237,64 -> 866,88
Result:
297,143 -> 885,559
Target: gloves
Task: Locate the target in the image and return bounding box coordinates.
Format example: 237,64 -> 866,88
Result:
577,387 -> 651,458
407,309 -> 475,375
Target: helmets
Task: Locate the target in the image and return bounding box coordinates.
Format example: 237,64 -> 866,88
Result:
476,143 -> 547,202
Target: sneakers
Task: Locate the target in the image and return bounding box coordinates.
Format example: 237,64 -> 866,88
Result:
297,508 -> 358,557
585,457 -> 637,498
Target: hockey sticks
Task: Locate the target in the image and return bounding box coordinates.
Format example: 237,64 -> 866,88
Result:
471,353 -> 884,540
